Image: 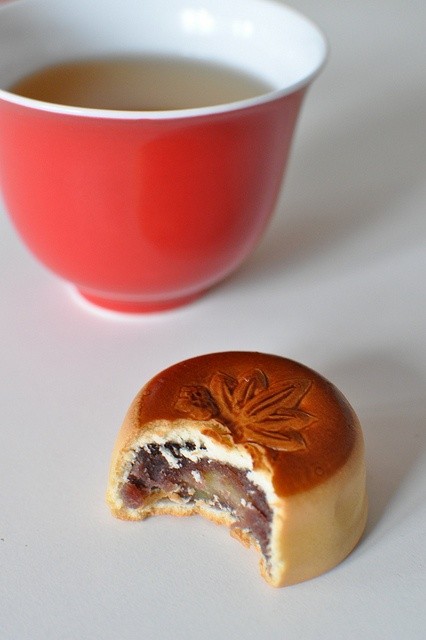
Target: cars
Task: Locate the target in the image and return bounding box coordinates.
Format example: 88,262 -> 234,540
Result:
0,1 -> 327,315
